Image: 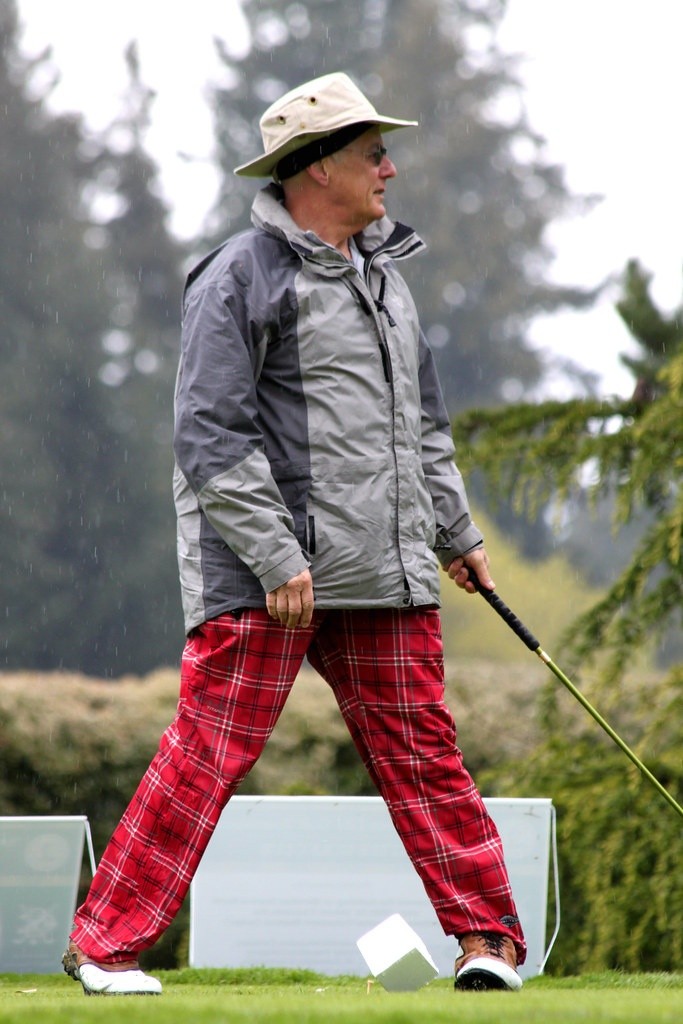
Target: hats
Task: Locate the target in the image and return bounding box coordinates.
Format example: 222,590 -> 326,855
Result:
234,72 -> 418,176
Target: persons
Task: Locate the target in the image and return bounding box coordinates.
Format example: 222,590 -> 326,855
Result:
65,70 -> 539,994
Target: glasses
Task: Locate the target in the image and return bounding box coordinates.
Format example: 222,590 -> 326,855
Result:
342,142 -> 388,166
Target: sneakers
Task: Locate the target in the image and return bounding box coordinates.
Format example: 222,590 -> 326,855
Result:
454,933 -> 523,990
62,939 -> 163,995
